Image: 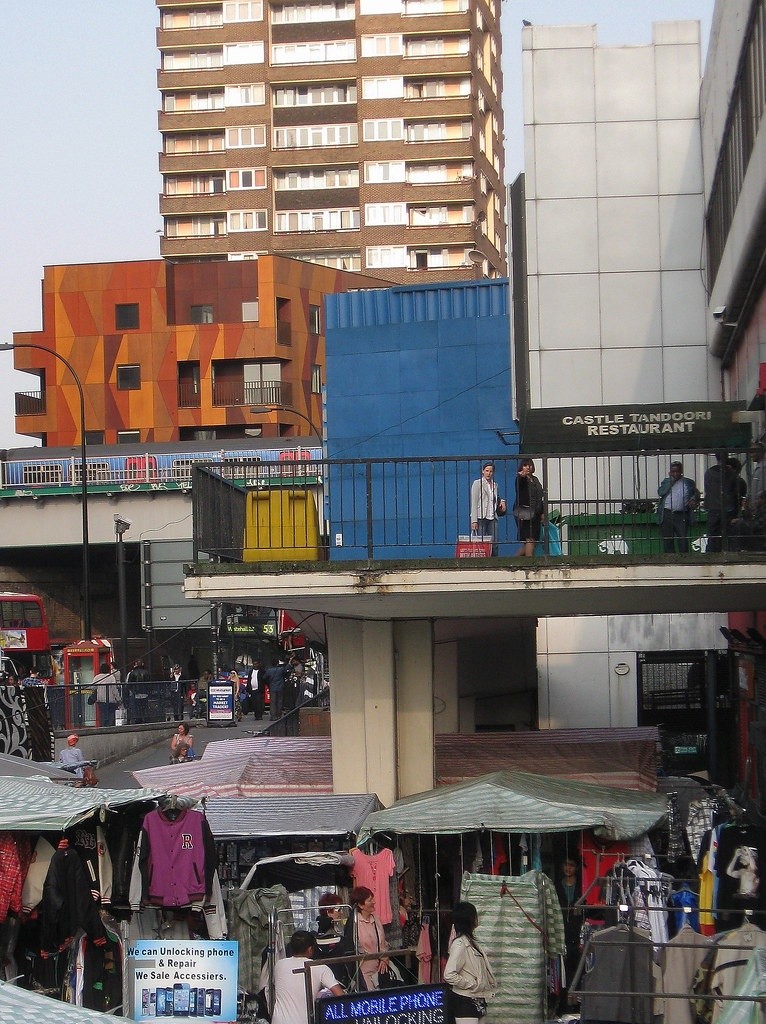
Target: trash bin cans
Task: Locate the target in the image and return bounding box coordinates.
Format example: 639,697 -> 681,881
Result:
546,509 -> 708,556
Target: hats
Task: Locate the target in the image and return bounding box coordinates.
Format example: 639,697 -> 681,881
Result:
67,735 -> 79,745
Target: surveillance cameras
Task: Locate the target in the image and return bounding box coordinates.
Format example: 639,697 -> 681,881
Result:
114,514 -> 132,527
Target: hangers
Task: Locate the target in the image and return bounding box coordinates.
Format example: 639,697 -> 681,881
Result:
614,904 -> 632,935
682,907 -> 692,930
608,853 -> 645,905
61,828 -> 66,842
163,795 -> 183,812
665,878 -> 701,903
742,908 -> 753,927
711,785 -> 755,830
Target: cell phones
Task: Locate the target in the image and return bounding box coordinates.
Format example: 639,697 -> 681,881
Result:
142,983 -> 222,1017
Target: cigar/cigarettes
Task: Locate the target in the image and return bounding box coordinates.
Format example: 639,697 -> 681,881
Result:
741,496 -> 746,498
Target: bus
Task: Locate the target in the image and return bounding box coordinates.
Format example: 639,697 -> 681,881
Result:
1,590 -> 56,693
64,637 -> 149,732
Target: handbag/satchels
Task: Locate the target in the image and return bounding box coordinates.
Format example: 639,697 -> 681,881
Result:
456,529 -> 493,557
115,705 -> 128,726
496,495 -> 507,517
513,505 -> 535,520
88,691 -> 97,705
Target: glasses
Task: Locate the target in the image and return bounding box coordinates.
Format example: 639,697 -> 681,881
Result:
335,908 -> 341,912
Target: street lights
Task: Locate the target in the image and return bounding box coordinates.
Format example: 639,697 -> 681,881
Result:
0,341 -> 92,643
250,406 -> 323,466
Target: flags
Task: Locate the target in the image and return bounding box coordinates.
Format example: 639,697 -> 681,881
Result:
277,609 -> 304,646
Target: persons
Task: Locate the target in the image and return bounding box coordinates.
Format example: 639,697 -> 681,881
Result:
7,675 -> 20,686
444,901 -> 497,1024
109,661 -> 126,709
557,852 -> 587,978
166,662 -> 188,721
349,886 -> 390,991
123,658 -> 152,724
270,930 -> 344,1024
512,458 -> 544,557
728,489 -> 766,552
169,741 -> 194,765
263,658 -> 291,721
311,891 -> 357,989
281,652 -> 303,710
704,451 -> 747,554
21,666 -> 42,685
91,662 -> 121,727
469,461 -> 508,556
187,668 -> 243,723
59,733 -> 86,780
170,723 -> 193,754
246,659 -> 266,721
657,461 -> 701,553
